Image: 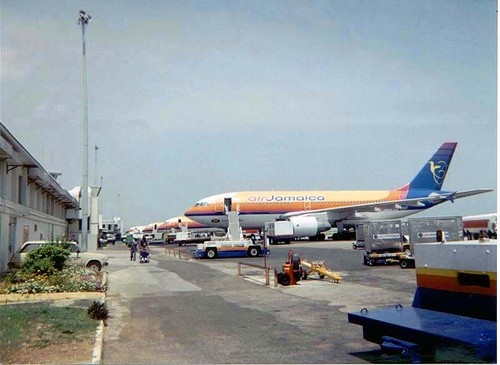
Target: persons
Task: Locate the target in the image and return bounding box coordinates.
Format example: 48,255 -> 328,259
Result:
128,238 -> 138,261
139,235 -> 149,263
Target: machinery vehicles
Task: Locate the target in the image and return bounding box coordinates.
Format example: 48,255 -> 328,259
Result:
348,217 -> 497,365
190,234 -> 271,259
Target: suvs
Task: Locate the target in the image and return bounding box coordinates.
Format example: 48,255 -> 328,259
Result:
7,240 -> 109,271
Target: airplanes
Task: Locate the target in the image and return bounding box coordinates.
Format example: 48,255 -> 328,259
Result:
122,142 -> 494,245
